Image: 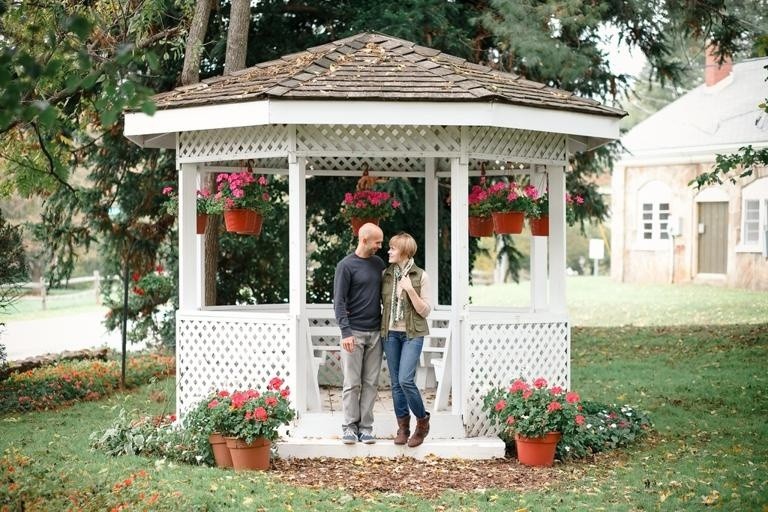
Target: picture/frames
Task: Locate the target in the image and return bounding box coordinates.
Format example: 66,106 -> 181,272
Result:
469,214 -> 494,237
529,216 -> 548,236
491,211 -> 523,234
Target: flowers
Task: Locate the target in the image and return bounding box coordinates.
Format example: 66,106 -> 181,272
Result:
484,372 -> 579,433
442,177 -> 585,217
189,379 -> 295,437
338,190 -> 398,219
160,170 -> 281,213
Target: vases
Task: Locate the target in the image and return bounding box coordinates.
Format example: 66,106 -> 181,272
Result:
351,217 -> 379,236
224,208 -> 247,232
227,437 -> 270,472
207,432 -> 231,469
511,428 -> 562,470
251,214 -> 264,235
196,213 -> 207,234
235,210 -> 254,235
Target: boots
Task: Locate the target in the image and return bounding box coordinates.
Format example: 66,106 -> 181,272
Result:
407,411 -> 431,448
393,414 -> 412,445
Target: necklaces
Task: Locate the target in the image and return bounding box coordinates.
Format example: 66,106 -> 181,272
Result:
391,255 -> 415,326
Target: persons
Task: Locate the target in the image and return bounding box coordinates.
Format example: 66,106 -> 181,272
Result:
379,230 -> 432,447
333,222 -> 386,445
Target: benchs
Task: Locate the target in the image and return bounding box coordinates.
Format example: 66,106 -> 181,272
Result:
306,307 -> 453,412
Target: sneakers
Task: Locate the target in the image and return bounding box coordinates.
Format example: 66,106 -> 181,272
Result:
357,431 -> 377,444
341,428 -> 358,444
